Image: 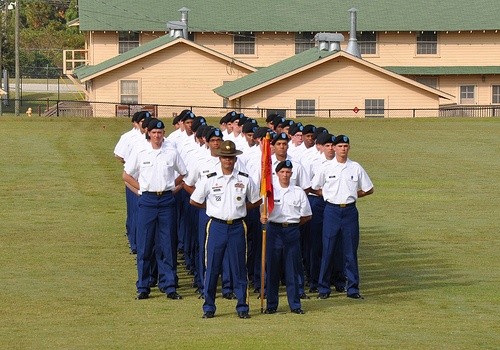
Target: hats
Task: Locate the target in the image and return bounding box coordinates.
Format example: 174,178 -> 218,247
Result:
172,109 -> 350,146
214,140 -> 243,156
276,160 -> 293,172
131,110 -> 164,132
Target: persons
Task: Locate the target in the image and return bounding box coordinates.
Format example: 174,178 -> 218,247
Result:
190,140 -> 262,319
311,135 -> 374,299
122,120 -> 187,299
114,109 -> 346,293
258,160 -> 313,314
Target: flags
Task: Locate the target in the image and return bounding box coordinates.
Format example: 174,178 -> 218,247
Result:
259,132 -> 275,219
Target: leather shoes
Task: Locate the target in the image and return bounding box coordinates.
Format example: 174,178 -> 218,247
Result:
199,294 -> 205,299
336,287 -> 347,292
135,293 -> 147,299
167,292 -> 182,299
224,293 -> 236,299
292,308 -> 304,314
303,266 -> 319,293
300,294 -> 309,299
257,295 -> 265,299
350,294 -> 364,299
265,308 -> 276,314
202,312 -> 213,318
238,311 -> 249,318
318,293 -> 328,299
177,249 -> 200,294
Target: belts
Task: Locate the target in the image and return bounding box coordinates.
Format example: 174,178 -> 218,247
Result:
270,222 -> 298,227
147,191 -> 170,195
328,202 -> 354,208
211,217 -> 242,224
